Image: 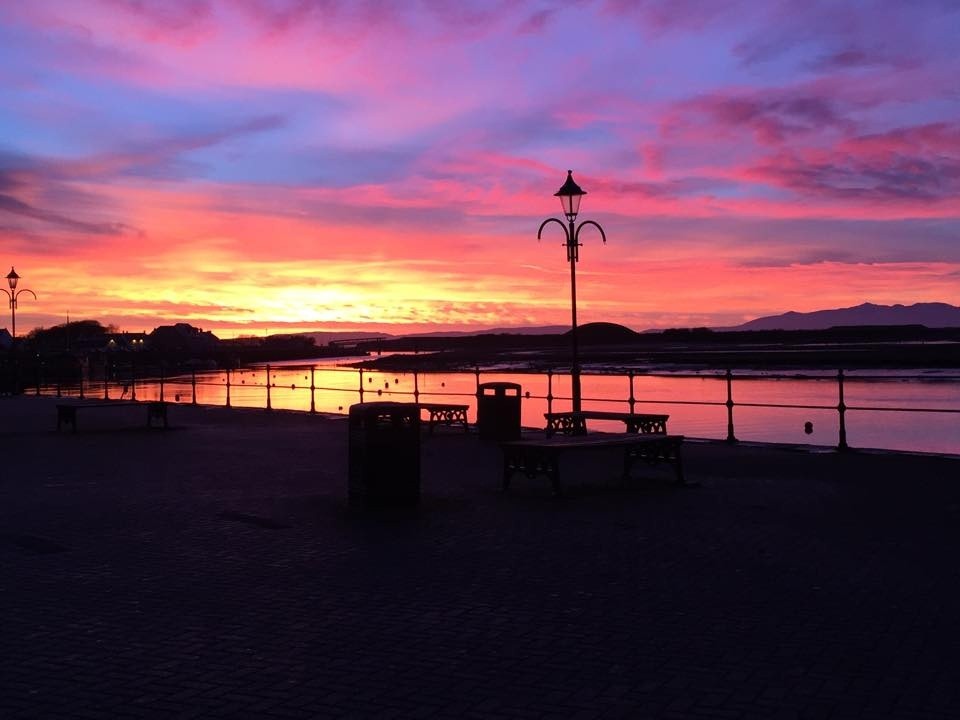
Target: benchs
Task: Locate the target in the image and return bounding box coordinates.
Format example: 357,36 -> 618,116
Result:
544,409 -> 670,440
401,402 -> 470,435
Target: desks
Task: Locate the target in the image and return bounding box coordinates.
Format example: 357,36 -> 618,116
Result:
493,434 -> 703,498
54,401 -> 177,434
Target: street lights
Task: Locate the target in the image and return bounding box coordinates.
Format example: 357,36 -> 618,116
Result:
0,266 -> 37,394
538,170 -> 607,436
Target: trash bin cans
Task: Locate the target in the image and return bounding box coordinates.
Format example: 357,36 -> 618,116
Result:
348,403 -> 421,528
475,382 -> 521,443
